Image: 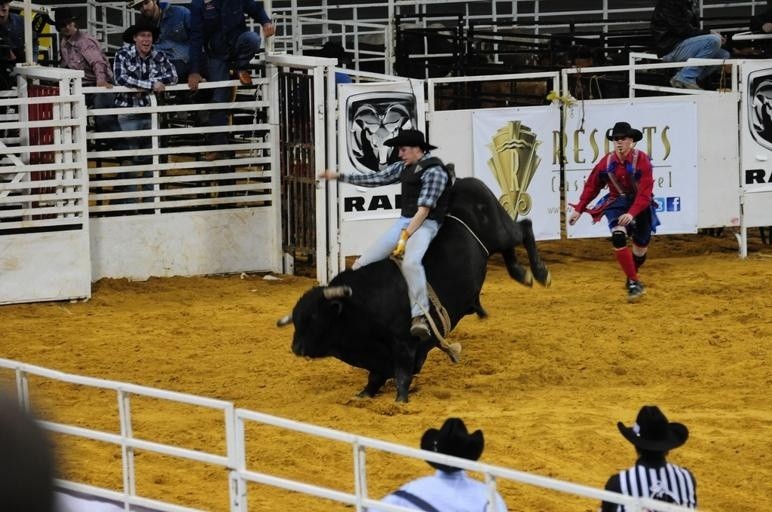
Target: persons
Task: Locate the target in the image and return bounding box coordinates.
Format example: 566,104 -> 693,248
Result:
651,3 -> 772,92
563,119 -> 655,294
600,407 -> 697,512
1,1 -> 274,208
552,46 -> 597,98
370,418 -> 509,512
1,395 -> 55,512
318,130 -> 449,337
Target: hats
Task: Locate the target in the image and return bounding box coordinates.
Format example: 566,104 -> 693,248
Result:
384,130 -> 437,150
123,22 -> 160,44
420,418 -> 484,472
605,122 -> 642,143
617,406 -> 689,453
43,10 -> 80,25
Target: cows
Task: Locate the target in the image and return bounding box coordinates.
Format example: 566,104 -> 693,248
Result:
277,175 -> 553,404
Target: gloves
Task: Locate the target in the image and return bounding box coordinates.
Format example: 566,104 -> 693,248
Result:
392,231 -> 410,256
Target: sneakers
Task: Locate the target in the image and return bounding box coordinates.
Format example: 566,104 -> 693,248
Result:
410,316 -> 431,341
625,276 -> 645,300
671,77 -> 699,90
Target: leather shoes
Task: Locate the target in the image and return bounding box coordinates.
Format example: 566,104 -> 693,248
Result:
237,70 -> 252,85
201,152 -> 218,160
